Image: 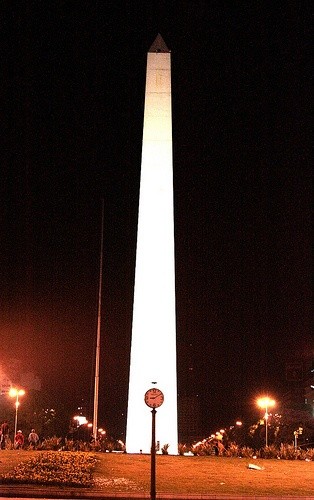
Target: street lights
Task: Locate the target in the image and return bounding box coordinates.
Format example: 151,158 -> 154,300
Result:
9,388 -> 24,435
256,396 -> 276,446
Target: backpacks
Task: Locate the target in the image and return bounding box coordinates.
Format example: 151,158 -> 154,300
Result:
30,433 -> 36,443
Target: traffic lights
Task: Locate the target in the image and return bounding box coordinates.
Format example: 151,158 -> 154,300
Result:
296,432 -> 298,438
299,427 -> 303,434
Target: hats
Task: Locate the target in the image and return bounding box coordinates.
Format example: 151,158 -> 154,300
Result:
18,430 -> 22,433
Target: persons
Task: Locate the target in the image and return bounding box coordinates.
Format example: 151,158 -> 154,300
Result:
14,430 -> 24,450
28,429 -> 39,450
0,420 -> 9,450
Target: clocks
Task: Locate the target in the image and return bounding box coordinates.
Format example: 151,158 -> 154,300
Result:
145,389 -> 164,406
